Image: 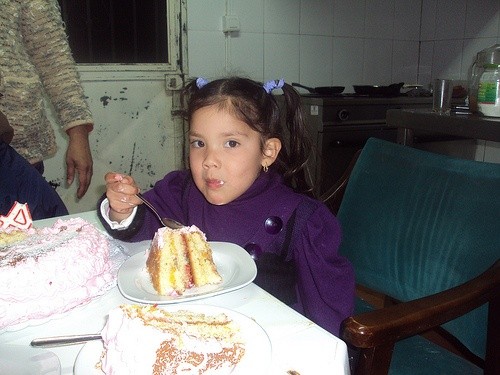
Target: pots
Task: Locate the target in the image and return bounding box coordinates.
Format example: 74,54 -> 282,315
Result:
292,82 -> 347,95
353,81 -> 404,96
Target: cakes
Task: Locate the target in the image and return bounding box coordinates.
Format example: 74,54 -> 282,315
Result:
0,202 -> 116,330
96,305 -> 247,375
146,223 -> 222,296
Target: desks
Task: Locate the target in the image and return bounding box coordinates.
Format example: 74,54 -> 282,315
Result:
385,107 -> 500,161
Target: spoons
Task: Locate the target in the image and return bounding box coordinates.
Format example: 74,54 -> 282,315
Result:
136,193 -> 183,230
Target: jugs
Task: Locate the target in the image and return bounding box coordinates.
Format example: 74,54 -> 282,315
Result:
467,47 -> 500,118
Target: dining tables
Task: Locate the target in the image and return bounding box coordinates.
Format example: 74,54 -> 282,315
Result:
0,209 -> 348,375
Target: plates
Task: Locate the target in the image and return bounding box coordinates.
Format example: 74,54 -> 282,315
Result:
74,305 -> 274,375
118,241 -> 259,302
0,343 -> 61,375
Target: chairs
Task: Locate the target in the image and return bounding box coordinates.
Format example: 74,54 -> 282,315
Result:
288,138 -> 500,375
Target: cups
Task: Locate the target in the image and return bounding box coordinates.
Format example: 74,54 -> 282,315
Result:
431,78 -> 454,115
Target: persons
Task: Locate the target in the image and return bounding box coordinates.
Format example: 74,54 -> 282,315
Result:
0,0 -> 94,201
97,75 -> 356,340
0,112 -> 69,221
